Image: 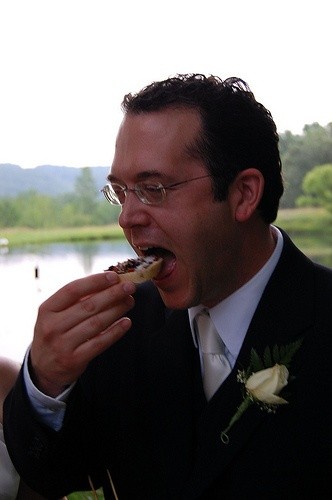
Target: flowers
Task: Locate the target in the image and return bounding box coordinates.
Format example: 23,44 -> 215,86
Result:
220,361 -> 291,447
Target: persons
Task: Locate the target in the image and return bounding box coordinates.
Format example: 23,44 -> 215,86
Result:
0,358 -> 22,498
5,73 -> 332,500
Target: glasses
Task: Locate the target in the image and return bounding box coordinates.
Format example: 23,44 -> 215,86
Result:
101,170 -> 233,206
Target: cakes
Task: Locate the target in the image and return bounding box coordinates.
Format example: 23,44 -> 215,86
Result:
104,255 -> 163,285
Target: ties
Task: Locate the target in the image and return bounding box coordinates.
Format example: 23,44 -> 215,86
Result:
193,311 -> 235,402
